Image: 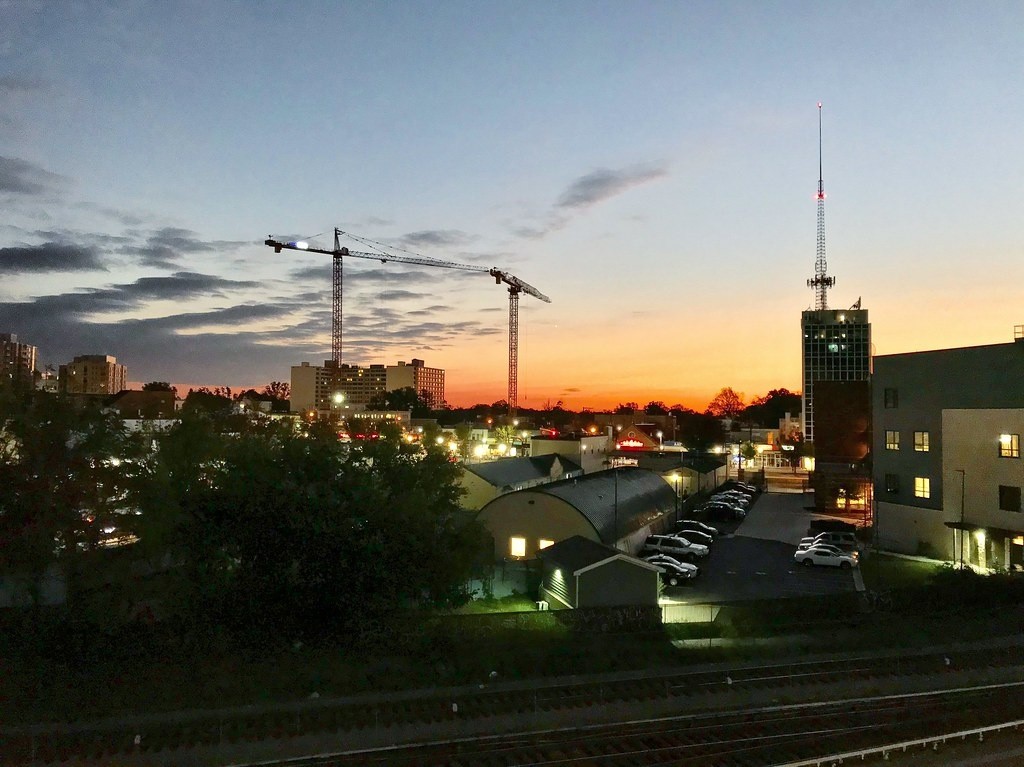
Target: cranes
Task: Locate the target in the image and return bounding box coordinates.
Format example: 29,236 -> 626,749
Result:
490,267 -> 553,413
264,226 -> 489,421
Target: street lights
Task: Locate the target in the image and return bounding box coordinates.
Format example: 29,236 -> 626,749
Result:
614,470 -> 618,549
739,439 -> 742,470
657,432 -> 662,450
956,469 -> 965,568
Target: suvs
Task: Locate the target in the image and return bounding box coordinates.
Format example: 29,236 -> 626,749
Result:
670,520 -> 718,536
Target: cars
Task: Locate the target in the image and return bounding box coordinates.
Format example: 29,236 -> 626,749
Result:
798,532 -> 857,548
806,544 -> 848,556
735,484 -> 755,494
692,490 -> 752,523
642,534 -> 709,564
798,539 -> 854,553
651,562 -> 691,587
794,548 -> 858,571
730,481 -> 756,490
644,556 -> 698,580
666,529 -> 713,544
